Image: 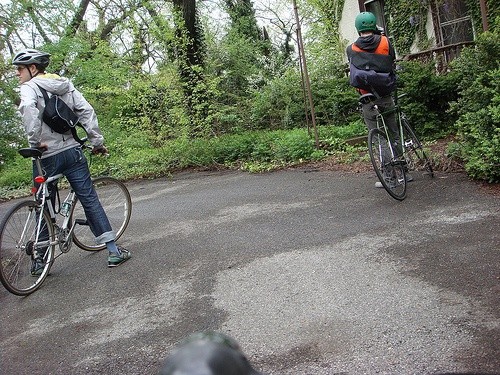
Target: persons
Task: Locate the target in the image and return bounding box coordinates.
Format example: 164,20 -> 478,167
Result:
345,12 -> 413,189
11,49 -> 132,277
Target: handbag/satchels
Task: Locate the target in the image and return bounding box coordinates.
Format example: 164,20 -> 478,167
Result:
349,49 -> 395,97
35,82 -> 89,145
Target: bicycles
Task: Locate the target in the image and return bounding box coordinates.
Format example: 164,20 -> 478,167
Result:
0,136 -> 134,297
358,83 -> 435,200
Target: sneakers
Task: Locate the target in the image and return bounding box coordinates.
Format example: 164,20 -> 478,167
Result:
30,258 -> 50,277
108,245 -> 131,266
375,168 -> 413,188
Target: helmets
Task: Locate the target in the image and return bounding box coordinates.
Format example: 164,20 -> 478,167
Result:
355,12 -> 376,35
12,49 -> 52,67
